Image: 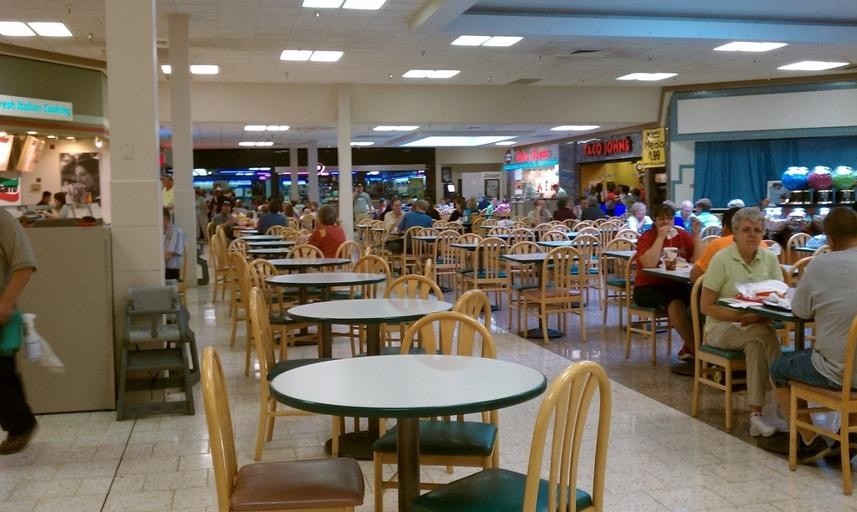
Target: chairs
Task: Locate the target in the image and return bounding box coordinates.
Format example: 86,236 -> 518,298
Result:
249,286 -> 350,461
787,317 -> 854,496
373,311 -> 500,511
691,273 -> 785,435
197,191 -> 855,365
200,345 -> 365,511
405,359 -> 613,511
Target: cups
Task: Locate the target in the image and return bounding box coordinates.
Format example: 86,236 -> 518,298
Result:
662,246 -> 679,271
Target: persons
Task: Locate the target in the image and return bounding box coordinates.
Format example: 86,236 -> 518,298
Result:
73,157 -> 99,204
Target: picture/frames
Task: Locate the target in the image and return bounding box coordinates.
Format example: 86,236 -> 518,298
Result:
484,179 -> 500,201
440,166 -> 452,183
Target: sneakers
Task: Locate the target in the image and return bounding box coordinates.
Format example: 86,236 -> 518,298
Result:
795,432 -> 831,463
676,347 -> 693,361
750,419 -> 775,438
769,413 -> 791,432
825,427 -> 856,464
1,420 -> 38,456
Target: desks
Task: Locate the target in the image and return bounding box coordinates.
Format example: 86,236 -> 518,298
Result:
270,355 -> 547,512
718,295 -> 818,455
287,298 -> 455,459
